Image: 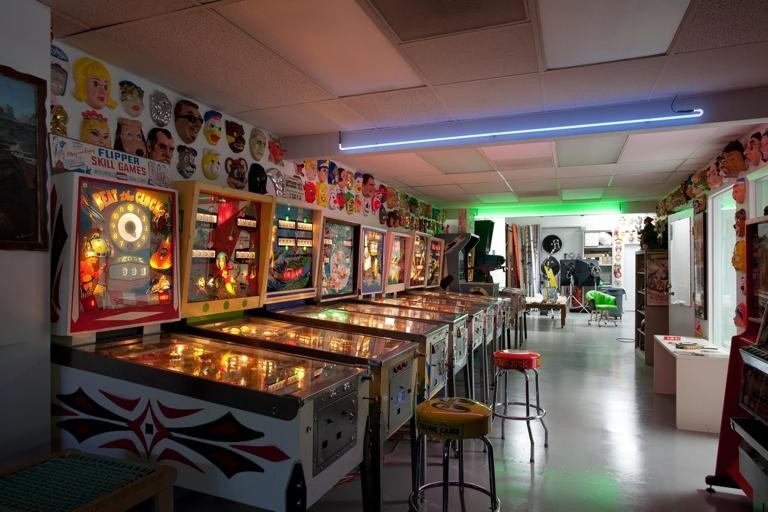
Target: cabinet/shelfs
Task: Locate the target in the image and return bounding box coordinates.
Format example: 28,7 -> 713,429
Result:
635,251 -> 669,366
583,230 -> 615,288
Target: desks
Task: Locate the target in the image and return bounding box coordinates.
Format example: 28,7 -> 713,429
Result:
654,334 -> 730,433
526,297 -> 567,329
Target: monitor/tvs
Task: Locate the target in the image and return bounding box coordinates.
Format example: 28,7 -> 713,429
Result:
70,173 -> 183,335
321,219 -> 356,299
388,233 -> 405,287
458,250 -> 467,281
362,229 -> 382,292
410,234 -> 426,290
187,190 -> 260,302
267,202 -> 315,294
427,238 -> 443,289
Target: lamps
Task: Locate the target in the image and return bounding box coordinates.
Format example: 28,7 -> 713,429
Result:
339,94 -> 705,151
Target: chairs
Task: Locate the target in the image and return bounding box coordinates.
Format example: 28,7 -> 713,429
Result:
585,290 -> 618,327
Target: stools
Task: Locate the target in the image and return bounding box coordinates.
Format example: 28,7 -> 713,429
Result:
492,349 -> 547,463
408,396 -> 501,512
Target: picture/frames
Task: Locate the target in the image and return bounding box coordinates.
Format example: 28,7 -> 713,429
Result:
0,64 -> 50,251
